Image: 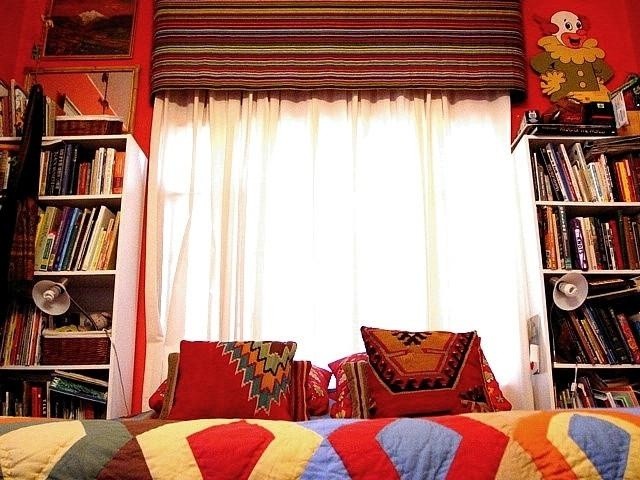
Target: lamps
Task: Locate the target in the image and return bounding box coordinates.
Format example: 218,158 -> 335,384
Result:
32,274 -> 102,332
548,269 -> 590,364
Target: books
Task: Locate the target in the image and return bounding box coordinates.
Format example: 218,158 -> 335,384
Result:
1,144 -> 125,196
1,370 -> 109,421
32,205 -> 120,271
1,78 -> 66,137
530,133 -> 640,411
1,288 -> 47,367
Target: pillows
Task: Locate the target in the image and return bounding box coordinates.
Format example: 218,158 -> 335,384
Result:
359,326 -> 486,420
167,339 -> 296,422
342,357 -> 493,422
328,347 -> 510,418
150,360 -> 332,415
160,352 -> 314,422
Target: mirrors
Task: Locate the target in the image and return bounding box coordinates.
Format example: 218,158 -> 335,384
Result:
22,65 -> 139,133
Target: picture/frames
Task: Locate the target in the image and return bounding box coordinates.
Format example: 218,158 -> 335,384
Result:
41,0 -> 138,61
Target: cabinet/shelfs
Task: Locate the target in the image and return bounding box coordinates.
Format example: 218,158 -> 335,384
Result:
0,134 -> 150,422
510,133 -> 640,411
